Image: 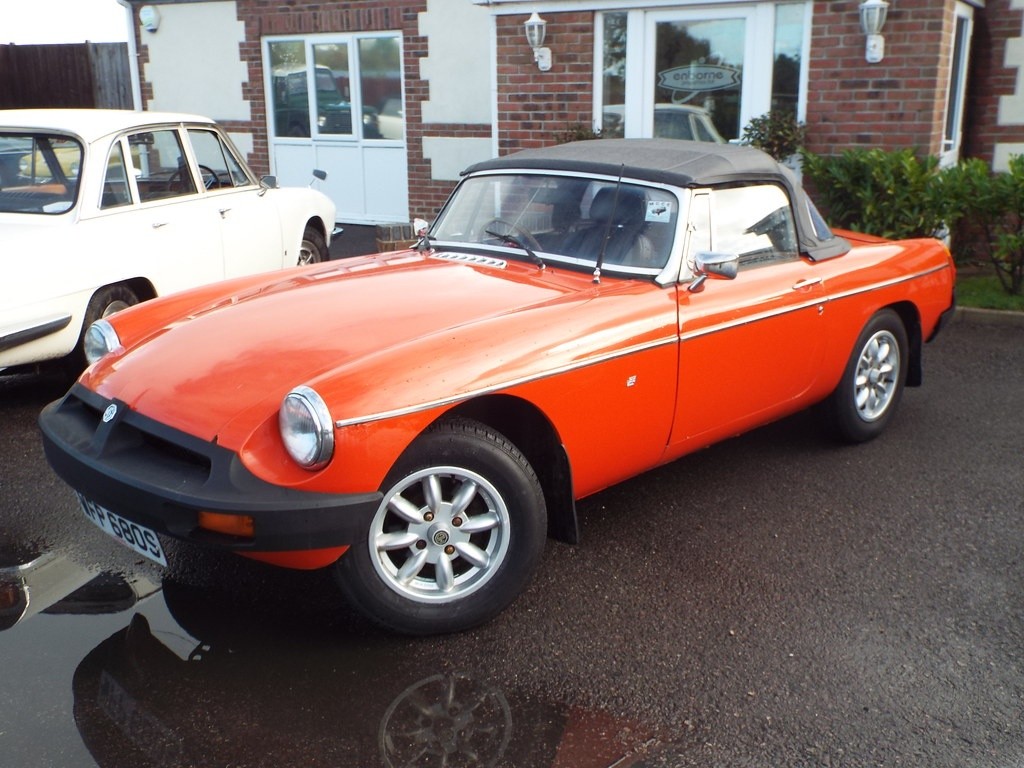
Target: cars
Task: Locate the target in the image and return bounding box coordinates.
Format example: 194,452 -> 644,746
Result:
34,138 -> 957,641
16,141 -> 142,183
273,62 -> 379,139
0,108 -> 338,369
604,104 -> 726,144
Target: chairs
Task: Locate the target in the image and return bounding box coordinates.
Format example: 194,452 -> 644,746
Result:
558,185 -> 653,268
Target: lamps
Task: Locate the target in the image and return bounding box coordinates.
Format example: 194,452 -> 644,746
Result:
858,0 -> 890,63
523,8 -> 552,71
